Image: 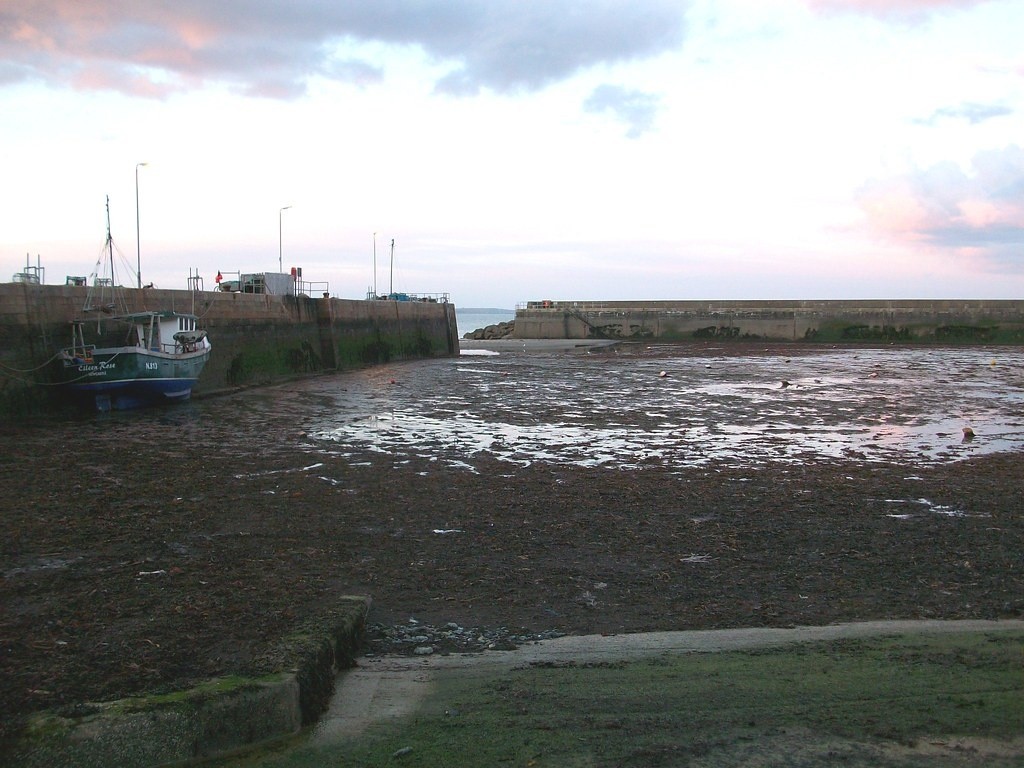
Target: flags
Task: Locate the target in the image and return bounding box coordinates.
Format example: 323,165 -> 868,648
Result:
216,271 -> 222,282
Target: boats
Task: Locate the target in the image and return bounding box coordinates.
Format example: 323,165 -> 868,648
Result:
55,194 -> 212,409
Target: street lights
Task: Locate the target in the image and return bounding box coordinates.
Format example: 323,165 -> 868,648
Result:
278,206 -> 290,273
